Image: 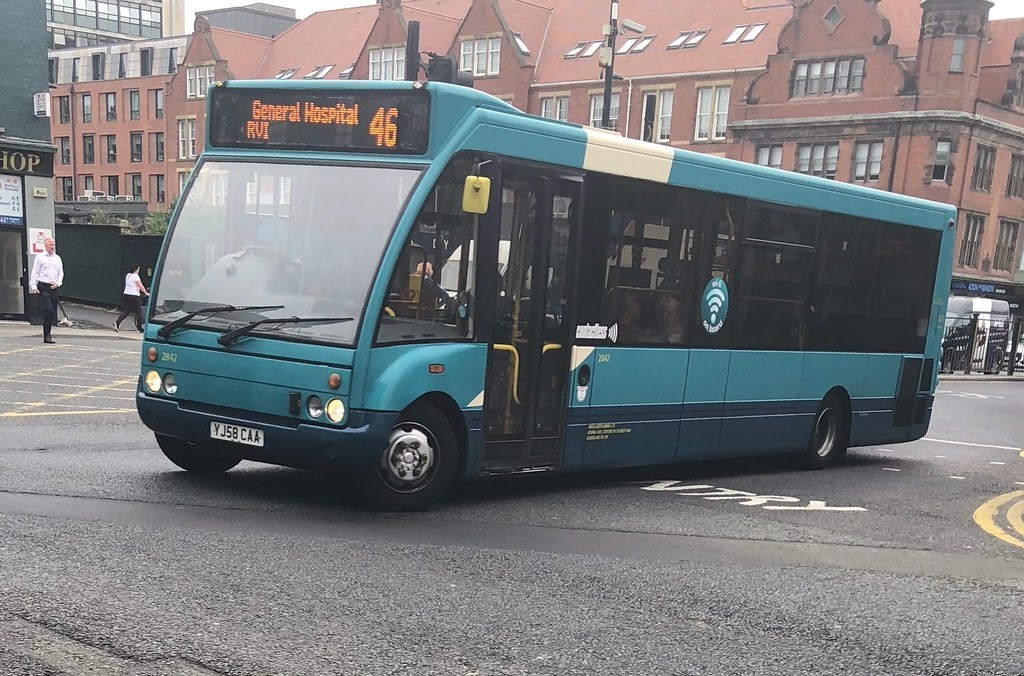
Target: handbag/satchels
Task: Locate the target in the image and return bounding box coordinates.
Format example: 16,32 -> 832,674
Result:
25,291 -> 53,325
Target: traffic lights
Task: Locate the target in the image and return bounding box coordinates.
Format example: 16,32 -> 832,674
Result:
423,51 -> 475,89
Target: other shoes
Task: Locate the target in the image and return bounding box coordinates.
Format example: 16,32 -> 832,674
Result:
44,334 -> 55,343
113,322 -> 119,332
138,327 -> 144,332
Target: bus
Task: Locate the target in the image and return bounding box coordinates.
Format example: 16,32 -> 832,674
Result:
135,77 -> 960,516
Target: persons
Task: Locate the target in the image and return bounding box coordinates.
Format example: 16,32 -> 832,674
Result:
615,292 -> 688,345
112,264 -> 151,333
412,262 -> 449,308
29,238 -> 67,342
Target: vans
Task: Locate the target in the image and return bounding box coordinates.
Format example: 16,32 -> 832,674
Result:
938,295 -> 1010,376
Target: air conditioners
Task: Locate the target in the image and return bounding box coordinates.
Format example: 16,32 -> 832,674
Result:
115,195 -> 134,202
96,196 -> 114,202
78,196 -> 95,201
